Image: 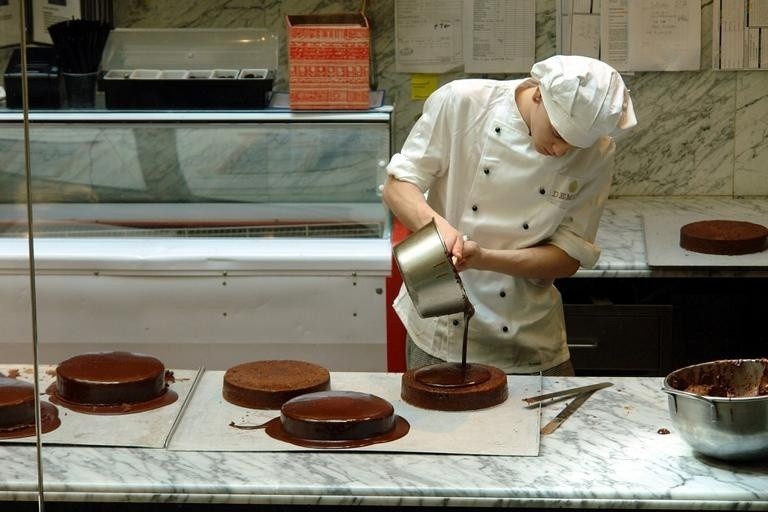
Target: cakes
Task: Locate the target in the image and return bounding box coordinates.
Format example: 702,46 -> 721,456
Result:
279,390 -> 396,442
0,374 -> 35,431
56,351 -> 166,408
401,362 -> 509,412
679,220 -> 768,255
222,359 -> 332,410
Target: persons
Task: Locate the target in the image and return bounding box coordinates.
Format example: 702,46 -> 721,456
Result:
380,53 -> 640,377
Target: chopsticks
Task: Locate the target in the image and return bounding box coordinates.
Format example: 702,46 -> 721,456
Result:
47,18 -> 112,74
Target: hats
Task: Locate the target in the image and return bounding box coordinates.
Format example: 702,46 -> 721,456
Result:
530,55 -> 637,149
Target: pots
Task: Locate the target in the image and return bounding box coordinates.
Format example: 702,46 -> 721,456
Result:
661,358 -> 768,466
392,217 -> 475,320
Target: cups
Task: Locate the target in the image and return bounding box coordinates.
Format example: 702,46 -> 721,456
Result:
61,68 -> 97,110
